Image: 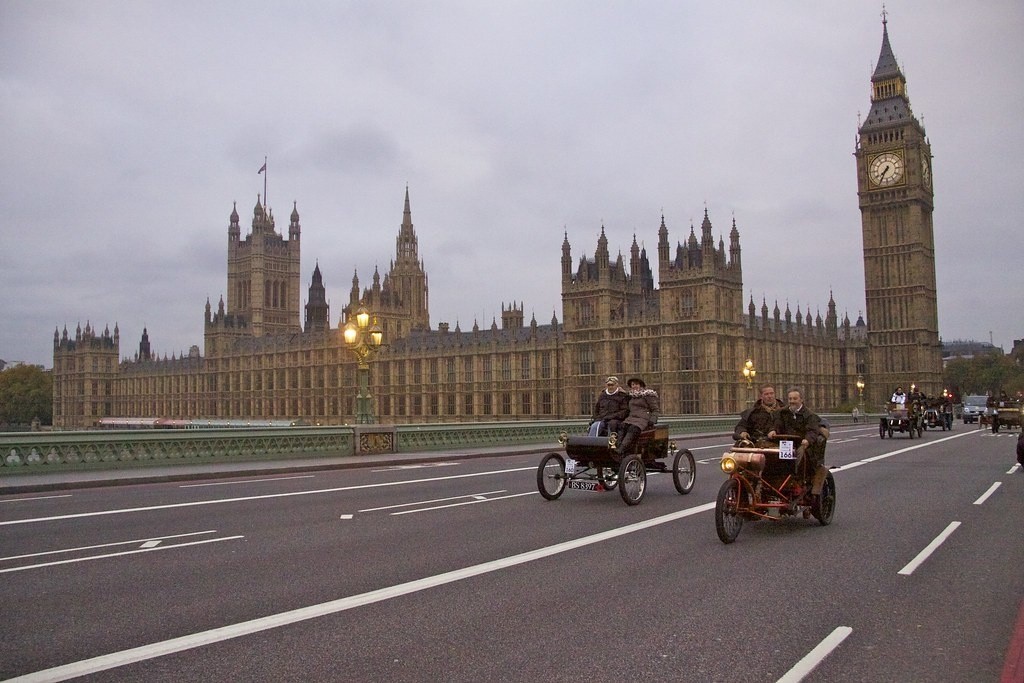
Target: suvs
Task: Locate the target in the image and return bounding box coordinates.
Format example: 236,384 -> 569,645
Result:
962,396 -> 990,424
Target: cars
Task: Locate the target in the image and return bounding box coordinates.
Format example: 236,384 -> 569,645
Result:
537,416 -> 696,506
879,402 -> 924,439
922,398 -> 954,432
991,402 -> 1024,435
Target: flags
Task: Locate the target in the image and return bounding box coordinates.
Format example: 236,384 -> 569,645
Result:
258,163 -> 266,175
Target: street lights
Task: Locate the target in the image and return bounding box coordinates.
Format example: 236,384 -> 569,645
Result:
857,376 -> 866,415
742,359 -> 756,409
943,388 -> 947,399
339,299 -> 384,423
910,381 -> 916,394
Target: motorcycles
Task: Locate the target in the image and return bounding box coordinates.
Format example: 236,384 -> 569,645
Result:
716,432 -> 842,543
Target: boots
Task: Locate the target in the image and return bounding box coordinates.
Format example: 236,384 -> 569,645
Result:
615,433 -> 635,456
616,434 -> 624,447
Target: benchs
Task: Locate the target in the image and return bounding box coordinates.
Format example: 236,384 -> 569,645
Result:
633,424 -> 670,452
812,419 -> 831,466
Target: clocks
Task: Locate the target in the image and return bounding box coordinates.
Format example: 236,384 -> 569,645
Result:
923,154 -> 931,186
867,153 -> 903,187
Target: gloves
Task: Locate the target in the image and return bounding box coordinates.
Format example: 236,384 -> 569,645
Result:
648,421 -> 654,425
603,416 -> 613,421
589,420 -> 595,426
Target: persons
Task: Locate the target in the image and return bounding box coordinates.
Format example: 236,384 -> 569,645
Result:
891,387 -> 949,416
588,376 -> 659,458
987,391 -> 1024,408
853,405 -> 858,423
732,384 -> 819,520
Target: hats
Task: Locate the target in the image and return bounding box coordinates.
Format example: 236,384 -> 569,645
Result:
627,376 -> 646,389
